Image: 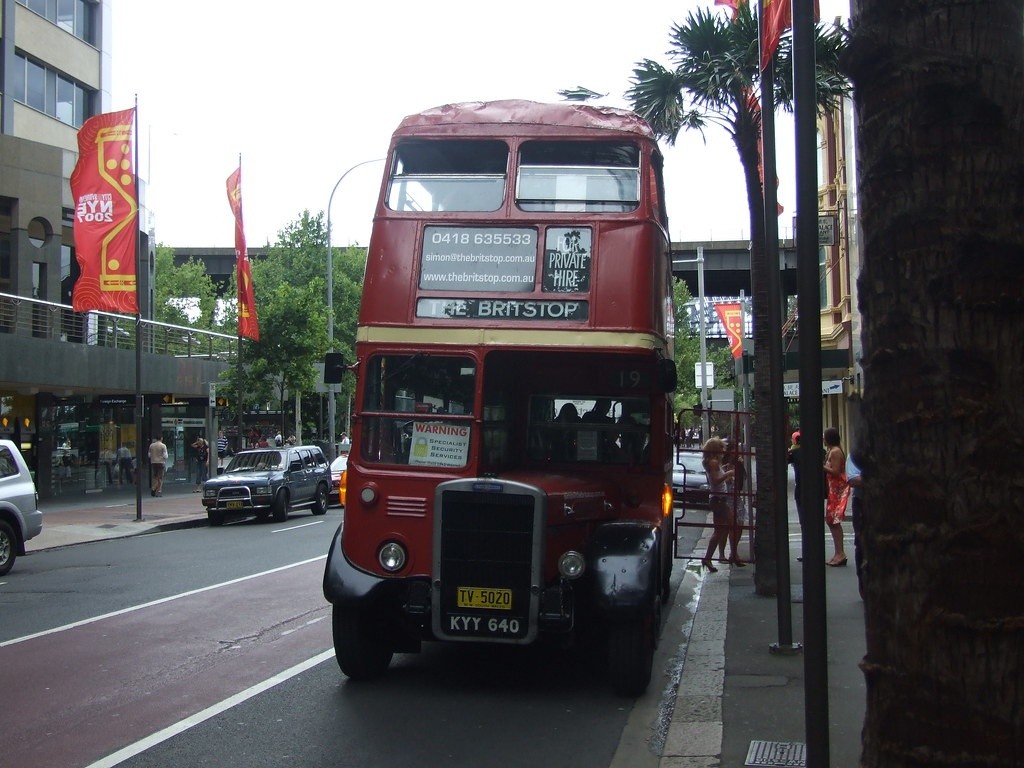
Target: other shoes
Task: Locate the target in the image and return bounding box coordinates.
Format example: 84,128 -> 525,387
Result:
151,489 -> 156,496
797,556 -> 802,561
158,491 -> 162,497
193,488 -> 202,493
719,560 -> 729,564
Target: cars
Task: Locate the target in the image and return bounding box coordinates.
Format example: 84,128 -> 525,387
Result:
328,451 -> 350,501
672,452 -> 709,505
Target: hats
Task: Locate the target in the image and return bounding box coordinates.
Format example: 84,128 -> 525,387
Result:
792,432 -> 801,444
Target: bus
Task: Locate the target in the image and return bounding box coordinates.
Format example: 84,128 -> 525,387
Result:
323,100 -> 679,692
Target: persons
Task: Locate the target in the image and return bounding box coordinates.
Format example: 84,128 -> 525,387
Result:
674,426 -> 703,448
148,433 -> 168,497
824,428 -> 850,567
845,453 -> 863,598
248,430 -> 297,449
788,427 -> 827,562
340,432 -> 349,444
548,397 -> 619,460
117,442 -> 132,484
191,430 -> 229,493
702,435 -> 746,572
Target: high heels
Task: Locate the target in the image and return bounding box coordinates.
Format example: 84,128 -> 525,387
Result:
729,556 -> 746,568
702,559 -> 717,572
825,555 -> 847,566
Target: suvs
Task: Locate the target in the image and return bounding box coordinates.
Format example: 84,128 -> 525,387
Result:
201,444 -> 333,527
0,439 -> 43,578
